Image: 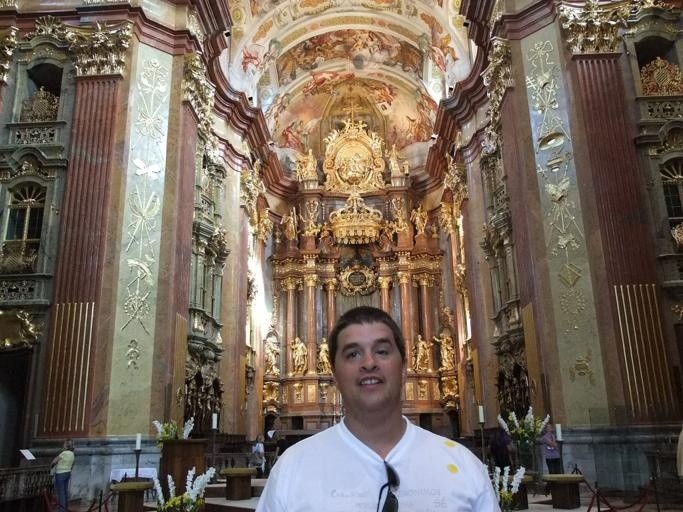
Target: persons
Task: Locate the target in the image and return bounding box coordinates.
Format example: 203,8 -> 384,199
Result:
270,432 -> 289,465
540,423 -> 562,498
48,438 -> 74,511
251,143 -> 456,373
253,431 -> 266,478
253,305 -> 502,511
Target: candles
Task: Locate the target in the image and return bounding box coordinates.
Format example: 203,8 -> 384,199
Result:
211,411 -> 218,428
477,404 -> 484,423
554,422 -> 563,442
134,431 -> 142,452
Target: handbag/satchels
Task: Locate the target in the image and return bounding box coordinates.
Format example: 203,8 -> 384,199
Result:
251,452 -> 263,465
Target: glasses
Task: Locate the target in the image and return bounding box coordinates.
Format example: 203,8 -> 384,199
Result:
377,460 -> 399,511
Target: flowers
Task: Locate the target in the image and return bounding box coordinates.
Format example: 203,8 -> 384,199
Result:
496,405 -> 550,460
151,415 -> 195,444
150,463 -> 216,512
484,459 -> 528,512
